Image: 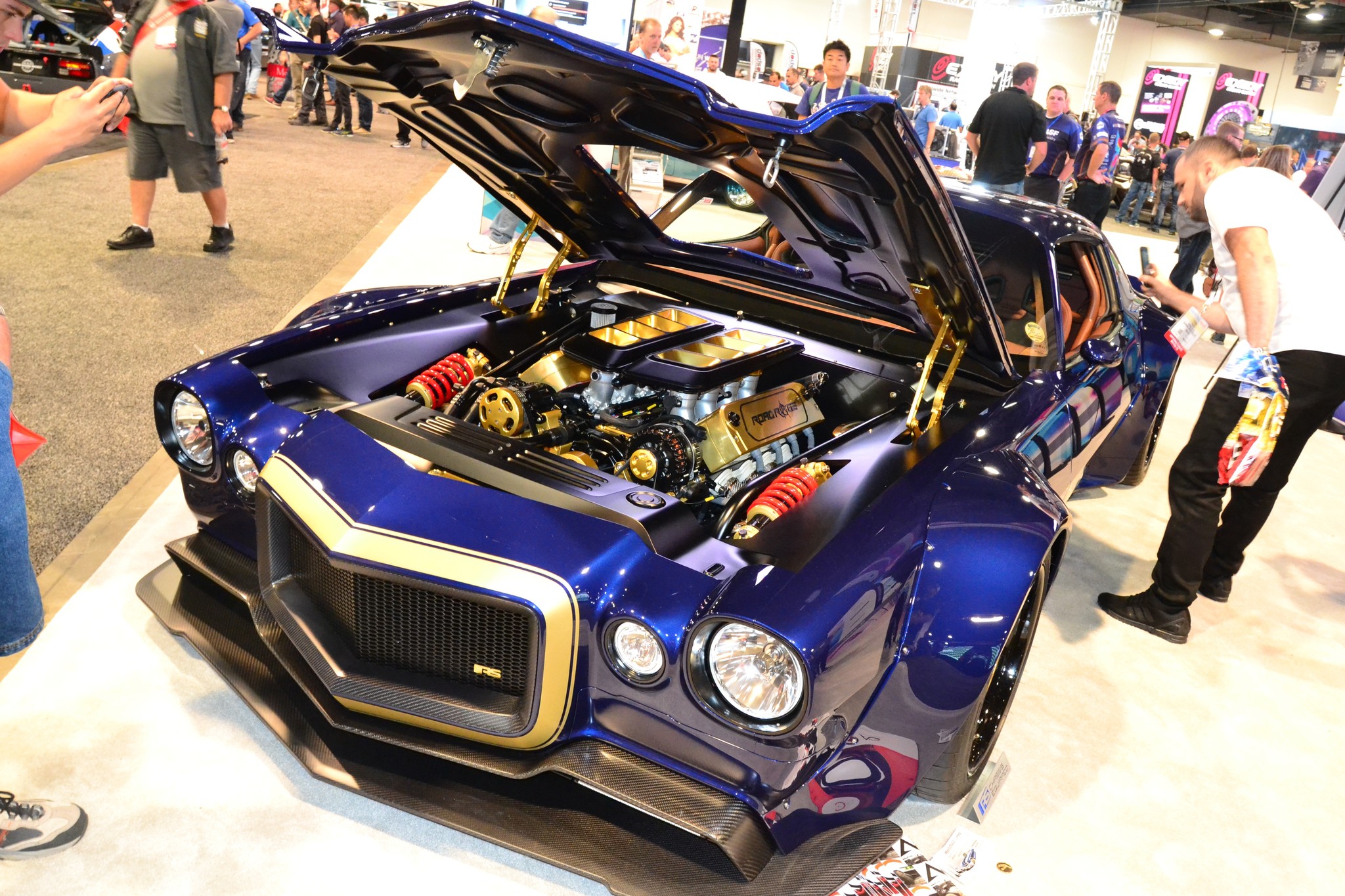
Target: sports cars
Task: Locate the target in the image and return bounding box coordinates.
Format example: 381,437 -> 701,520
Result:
2,1 -> 132,96
133,0 -> 1187,896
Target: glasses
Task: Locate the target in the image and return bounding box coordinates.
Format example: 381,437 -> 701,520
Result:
1227,135 -> 1243,143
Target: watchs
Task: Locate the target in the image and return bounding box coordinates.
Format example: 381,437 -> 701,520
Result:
213,106 -> 229,113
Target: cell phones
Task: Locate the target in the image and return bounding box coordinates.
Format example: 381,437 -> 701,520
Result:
98,82 -> 130,112
1140,246 -> 1152,288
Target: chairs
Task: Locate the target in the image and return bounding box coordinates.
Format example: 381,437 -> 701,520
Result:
28,20 -> 67,43
980,253 -> 1074,354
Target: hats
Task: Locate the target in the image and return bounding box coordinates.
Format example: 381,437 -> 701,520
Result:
1179,131 -> 1190,140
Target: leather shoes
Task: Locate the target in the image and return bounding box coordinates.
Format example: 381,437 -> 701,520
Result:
203,224 -> 234,252
107,225 -> 155,249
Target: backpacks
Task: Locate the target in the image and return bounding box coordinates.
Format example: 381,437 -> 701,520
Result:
1129,151 -> 1153,183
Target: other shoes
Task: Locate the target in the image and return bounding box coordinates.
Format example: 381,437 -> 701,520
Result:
225,120 -> 243,143
288,112 -> 329,126
246,93 -> 260,99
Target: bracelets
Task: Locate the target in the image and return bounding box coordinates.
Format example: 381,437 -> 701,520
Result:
1056,177 -> 1066,185
925,145 -> 931,148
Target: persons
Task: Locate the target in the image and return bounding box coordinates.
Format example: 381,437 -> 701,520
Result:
1068,81 -> 1126,231
702,55 -> 727,75
662,44 -> 673,61
615,18 -> 662,193
913,85 -> 938,156
0,0 -> 91,855
628,34 -> 639,54
735,63 -> 825,97
1058,109 -> 1340,346
663,15 -> 689,56
1097,136 -> 1345,646
939,103 -> 963,133
106,1 -> 235,251
95,0 -> 439,161
890,89 -> 901,99
1024,85 -> 1079,203
794,41 -> 869,121
965,62 -> 1047,196
465,6 -> 562,254
650,41 -> 667,63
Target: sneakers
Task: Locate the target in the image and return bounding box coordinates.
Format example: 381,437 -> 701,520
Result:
0,791 -> 88,860
331,126 -> 353,137
421,137 -> 430,148
1198,573 -> 1232,602
1098,583 -> 1191,644
263,96 -> 281,109
391,139 -> 410,148
1147,227 -> 1159,233
1128,221 -> 1140,228
321,126 -> 339,133
1169,231 -> 1175,235
1116,219 -> 1122,223
1210,330 -> 1225,345
352,126 -> 371,134
